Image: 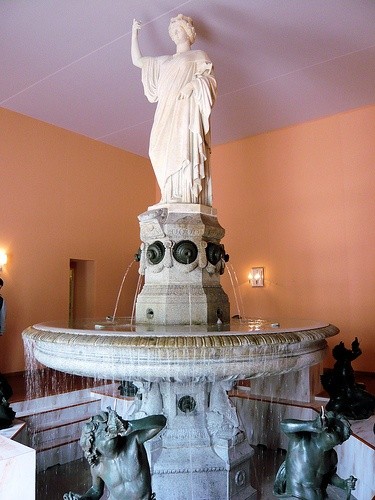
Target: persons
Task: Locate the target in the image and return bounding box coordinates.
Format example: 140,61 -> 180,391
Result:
130,13 -> 217,206
63,406 -> 167,500
0,278 -> 6,335
280,406 -> 358,500
331,336 -> 369,403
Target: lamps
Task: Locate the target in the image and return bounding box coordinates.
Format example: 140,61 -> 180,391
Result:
248,268 -> 264,287
